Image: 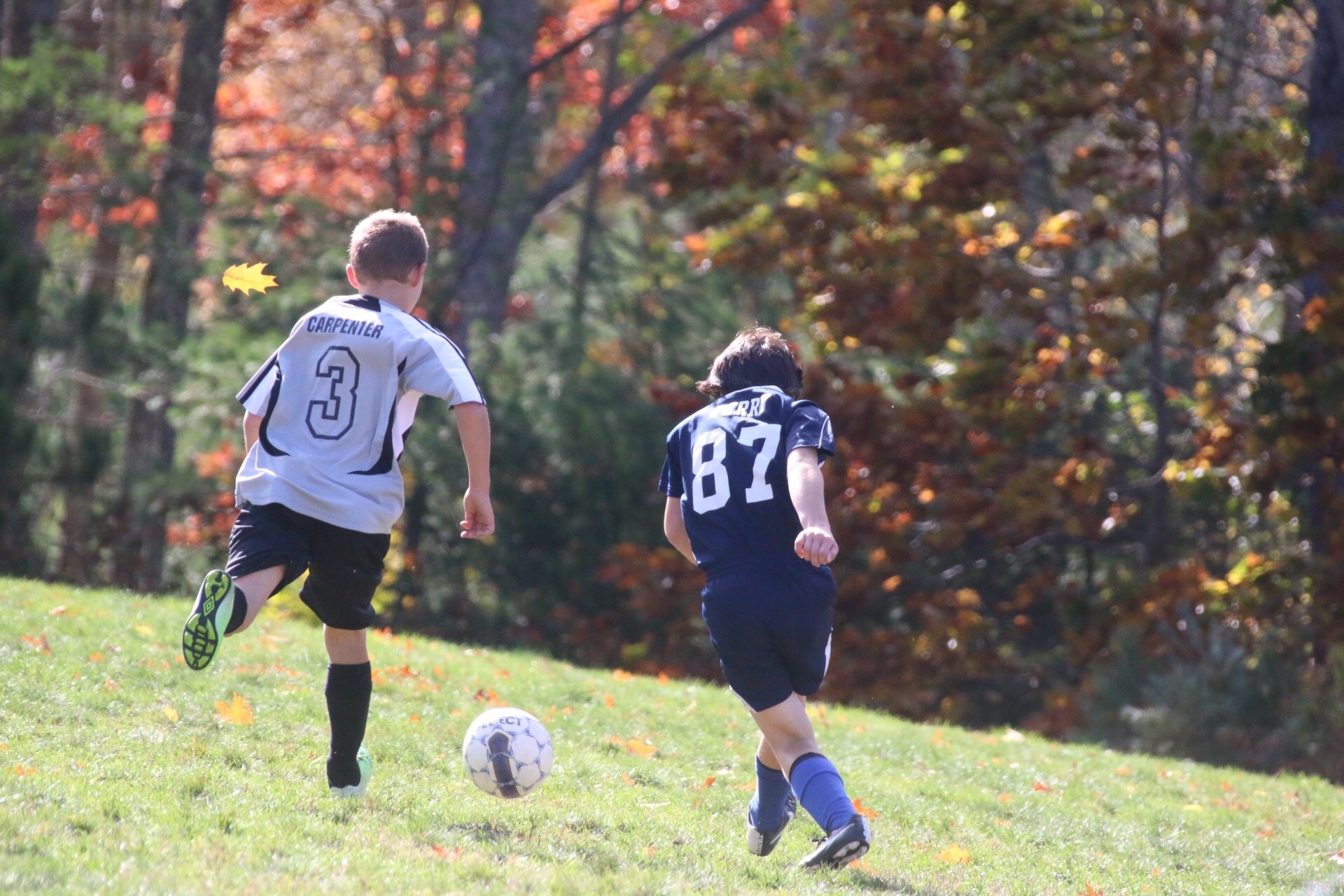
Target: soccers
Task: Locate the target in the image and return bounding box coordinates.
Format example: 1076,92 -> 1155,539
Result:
461,706 -> 551,802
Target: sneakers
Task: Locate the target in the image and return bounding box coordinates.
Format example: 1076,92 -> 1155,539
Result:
745,793 -> 797,856
799,812 -> 870,874
180,570 -> 239,671
310,743 -> 371,799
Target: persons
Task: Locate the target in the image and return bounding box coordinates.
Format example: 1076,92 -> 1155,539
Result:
658,326 -> 871,875
182,208 -> 495,798
